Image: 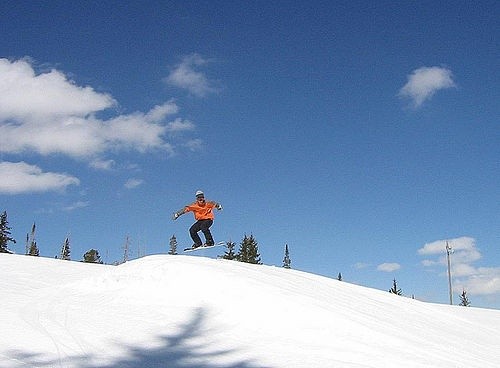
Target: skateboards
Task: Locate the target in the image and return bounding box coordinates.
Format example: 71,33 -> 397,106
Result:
183,242 -> 225,252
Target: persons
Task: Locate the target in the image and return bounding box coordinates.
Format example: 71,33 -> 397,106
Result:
173,189 -> 224,249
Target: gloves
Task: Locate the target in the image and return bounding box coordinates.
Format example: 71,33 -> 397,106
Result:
171,212 -> 179,220
218,204 -> 224,211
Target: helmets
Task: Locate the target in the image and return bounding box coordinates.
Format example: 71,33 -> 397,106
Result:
195,191 -> 204,196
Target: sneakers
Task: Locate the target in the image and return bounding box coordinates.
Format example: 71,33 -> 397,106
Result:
203,242 -> 215,247
192,244 -> 203,249
